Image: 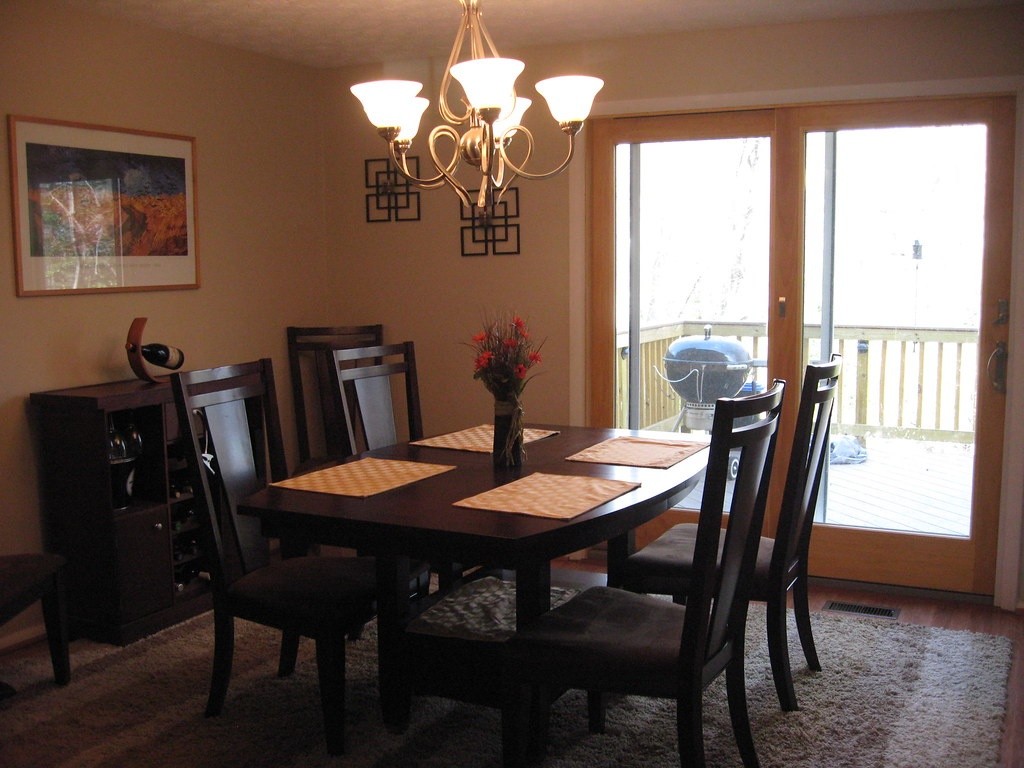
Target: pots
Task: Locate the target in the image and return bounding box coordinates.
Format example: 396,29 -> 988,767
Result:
662,325 -> 768,402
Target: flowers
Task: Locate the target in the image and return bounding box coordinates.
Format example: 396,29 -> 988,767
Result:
493,399 -> 523,468
466,307 -> 544,400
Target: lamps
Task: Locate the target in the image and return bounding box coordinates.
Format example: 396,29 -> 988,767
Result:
350,1 -> 605,230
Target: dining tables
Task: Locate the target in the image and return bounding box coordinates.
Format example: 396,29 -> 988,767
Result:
232,419 -> 711,768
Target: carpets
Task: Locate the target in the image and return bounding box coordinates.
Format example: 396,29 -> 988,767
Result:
0,545 -> 1013,768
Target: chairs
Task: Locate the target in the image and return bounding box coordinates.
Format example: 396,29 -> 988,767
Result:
0,553 -> 71,699
285,325 -> 386,480
326,341 -> 473,599
170,358 -> 430,757
495,379 -> 789,768
623,353 -> 842,713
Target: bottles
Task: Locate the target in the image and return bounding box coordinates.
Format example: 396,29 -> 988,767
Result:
125,343 -> 184,369
168,479 -> 211,592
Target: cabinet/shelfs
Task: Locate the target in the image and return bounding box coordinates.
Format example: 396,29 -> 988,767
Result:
28,357 -> 291,646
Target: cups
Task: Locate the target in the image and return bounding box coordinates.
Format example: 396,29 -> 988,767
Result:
126,424 -> 144,457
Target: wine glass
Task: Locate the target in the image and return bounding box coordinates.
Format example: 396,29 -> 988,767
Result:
109,414 -> 127,460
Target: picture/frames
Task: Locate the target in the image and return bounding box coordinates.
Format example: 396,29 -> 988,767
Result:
5,113 -> 200,297
